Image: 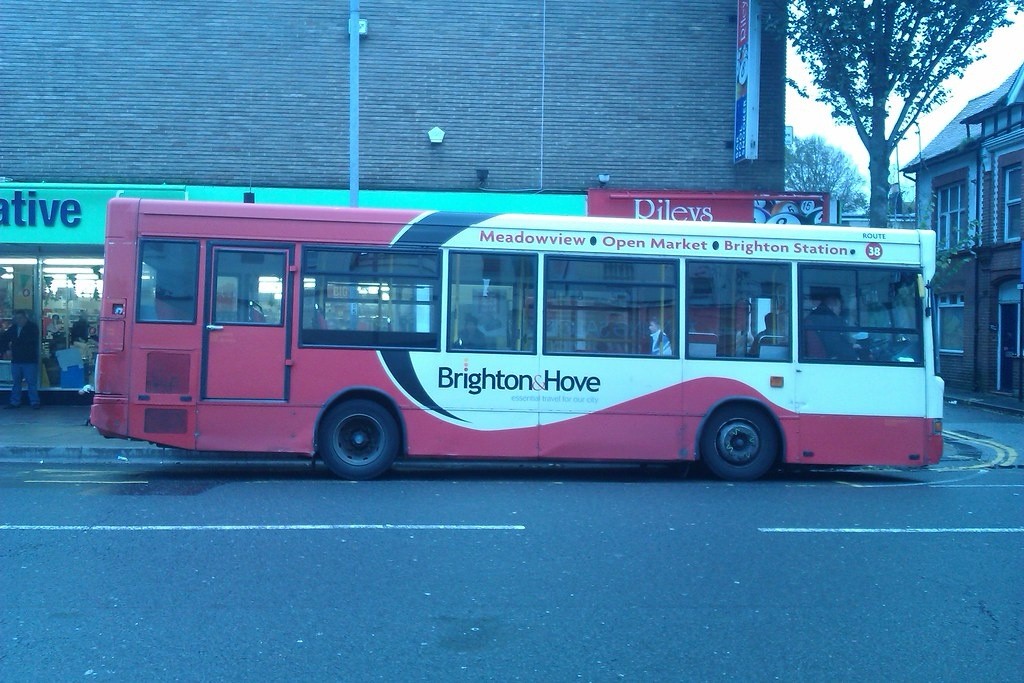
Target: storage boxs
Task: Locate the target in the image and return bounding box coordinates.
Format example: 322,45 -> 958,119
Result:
60,363 -> 90,389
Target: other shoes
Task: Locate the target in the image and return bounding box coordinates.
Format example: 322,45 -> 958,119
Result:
32,404 -> 40,409
4,404 -> 20,409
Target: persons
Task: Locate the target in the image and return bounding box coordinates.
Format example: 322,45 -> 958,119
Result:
737,328 -> 753,356
749,313 -> 774,357
71,311 -> 99,346
0,310 -> 40,409
803,293 -> 861,360
648,316 -> 672,356
453,315 -> 486,350
599,314 -> 626,353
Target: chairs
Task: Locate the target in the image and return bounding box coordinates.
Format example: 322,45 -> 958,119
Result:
757,335 -> 789,359
246,300 -> 265,323
311,304 -> 328,331
805,330 -> 828,358
688,332 -> 719,358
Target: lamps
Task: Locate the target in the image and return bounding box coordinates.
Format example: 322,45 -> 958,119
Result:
474,169 -> 489,183
598,173 -> 610,189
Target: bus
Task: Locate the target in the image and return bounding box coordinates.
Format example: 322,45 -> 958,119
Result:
80,196 -> 946,484
